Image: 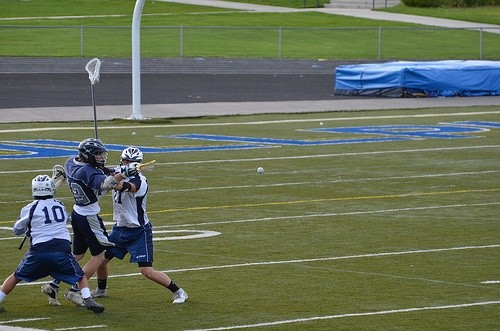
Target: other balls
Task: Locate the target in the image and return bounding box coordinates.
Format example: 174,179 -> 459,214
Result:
257,166 -> 264,174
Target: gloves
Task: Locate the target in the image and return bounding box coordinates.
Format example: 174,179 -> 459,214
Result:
121,162 -> 140,177
103,175 -> 119,189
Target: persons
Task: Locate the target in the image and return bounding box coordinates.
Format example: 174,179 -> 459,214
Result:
90,145 -> 189,305
40,138 -> 139,308
0,175 -> 105,314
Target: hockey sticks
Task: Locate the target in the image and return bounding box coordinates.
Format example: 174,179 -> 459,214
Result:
84,56 -> 103,144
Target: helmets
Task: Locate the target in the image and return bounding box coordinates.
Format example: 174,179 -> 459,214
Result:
31,174 -> 56,196
78,138 -> 108,167
120,147 -> 143,163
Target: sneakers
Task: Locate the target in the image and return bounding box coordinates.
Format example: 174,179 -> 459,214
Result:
90,286 -> 108,297
173,288 -> 188,303
41,283 -> 61,305
83,297 -> 104,313
64,289 -> 85,307
